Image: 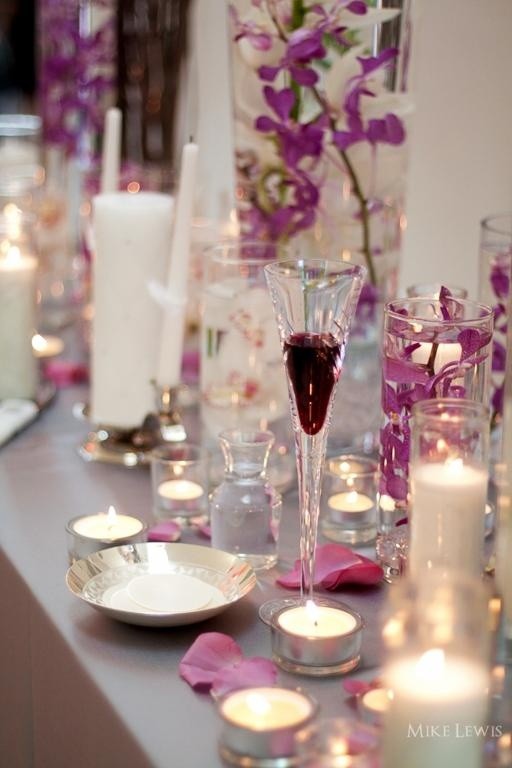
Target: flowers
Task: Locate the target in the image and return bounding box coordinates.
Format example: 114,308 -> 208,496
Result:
39,0 -> 122,193
231,0 -> 407,308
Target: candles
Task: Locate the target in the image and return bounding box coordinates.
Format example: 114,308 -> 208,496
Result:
0,104 -> 512,768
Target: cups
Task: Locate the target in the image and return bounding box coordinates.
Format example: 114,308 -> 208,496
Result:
1,108 -> 512,768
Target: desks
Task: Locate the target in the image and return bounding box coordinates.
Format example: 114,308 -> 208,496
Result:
0,239 -> 511,768
